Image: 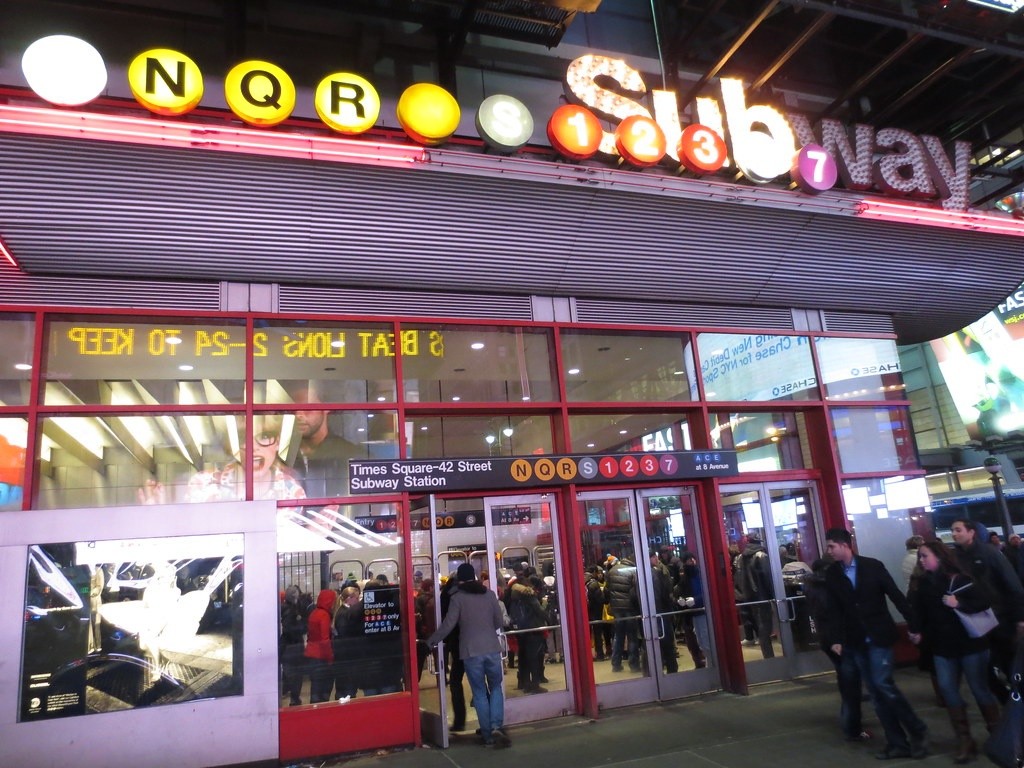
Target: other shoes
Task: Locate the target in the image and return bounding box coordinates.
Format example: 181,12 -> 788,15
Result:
545,653 -> 564,664
845,728 -> 875,741
476,728 -> 482,735
606,652 -> 612,659
631,667 -> 641,672
524,682 -> 548,693
911,724 -> 928,759
449,725 -> 465,731
741,639 -> 754,646
517,679 -> 530,689
480,738 -> 496,746
876,741 -> 911,759
937,697 -> 967,708
755,641 -> 760,645
613,665 -> 623,672
491,728 -> 512,747
538,675 -> 548,682
597,654 -> 609,660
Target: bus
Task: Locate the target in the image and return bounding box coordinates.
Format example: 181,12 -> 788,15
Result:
932,485 -> 1024,550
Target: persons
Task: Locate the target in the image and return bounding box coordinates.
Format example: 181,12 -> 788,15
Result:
140,386 -> 373,541
87,566 -> 104,654
806,517 -> 1024,768
279,571 -> 405,704
412,561 -> 565,700
427,562 -> 510,745
582,532 -> 811,677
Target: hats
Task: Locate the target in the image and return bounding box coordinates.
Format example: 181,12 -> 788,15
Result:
457,563 -> 475,581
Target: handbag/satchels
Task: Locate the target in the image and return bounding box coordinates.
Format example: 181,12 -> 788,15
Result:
985,692 -> 1024,768
948,573 -> 999,638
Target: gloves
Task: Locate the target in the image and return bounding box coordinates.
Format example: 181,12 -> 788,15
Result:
677,597 -> 686,606
685,597 -> 695,607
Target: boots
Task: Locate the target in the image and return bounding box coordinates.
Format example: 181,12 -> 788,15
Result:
983,704 -> 1000,735
951,719 -> 977,764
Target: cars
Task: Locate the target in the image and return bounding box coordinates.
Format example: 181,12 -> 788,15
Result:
88,557 -> 244,632
